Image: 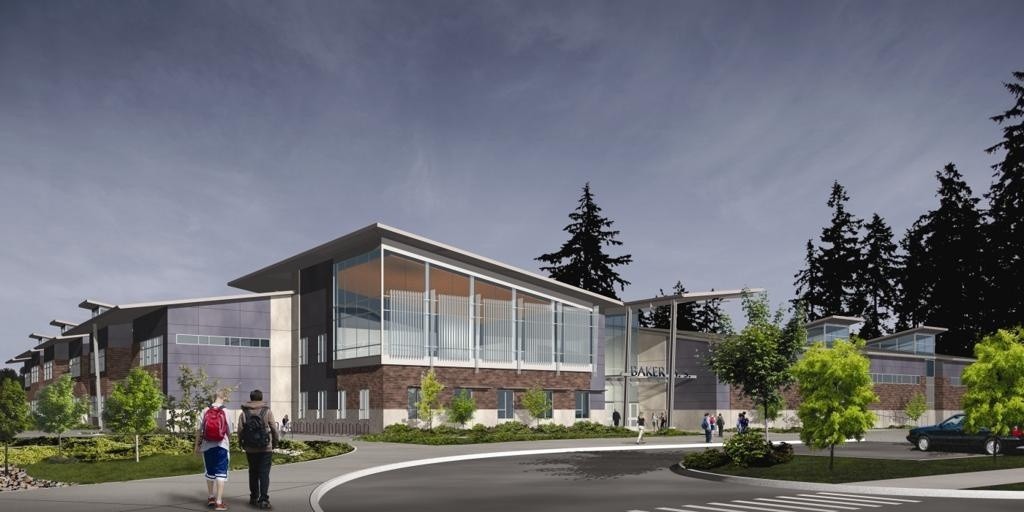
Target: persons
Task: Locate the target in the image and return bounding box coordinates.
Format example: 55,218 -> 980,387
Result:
280,413 -> 289,436
237,390 -> 283,511
195,388 -> 233,512
610,404 -> 751,447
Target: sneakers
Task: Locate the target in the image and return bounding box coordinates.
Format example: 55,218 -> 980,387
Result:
260,500 -> 271,508
215,503 -> 228,510
208,496 -> 215,506
250,497 -> 259,505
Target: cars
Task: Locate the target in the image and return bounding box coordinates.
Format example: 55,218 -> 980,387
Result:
905,412 -> 1024,455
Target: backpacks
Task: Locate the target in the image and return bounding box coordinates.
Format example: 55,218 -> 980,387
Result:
241,407 -> 269,448
701,418 -> 710,429
201,405 -> 228,442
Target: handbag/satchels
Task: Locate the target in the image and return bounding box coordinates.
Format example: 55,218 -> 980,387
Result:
717,419 -> 722,426
